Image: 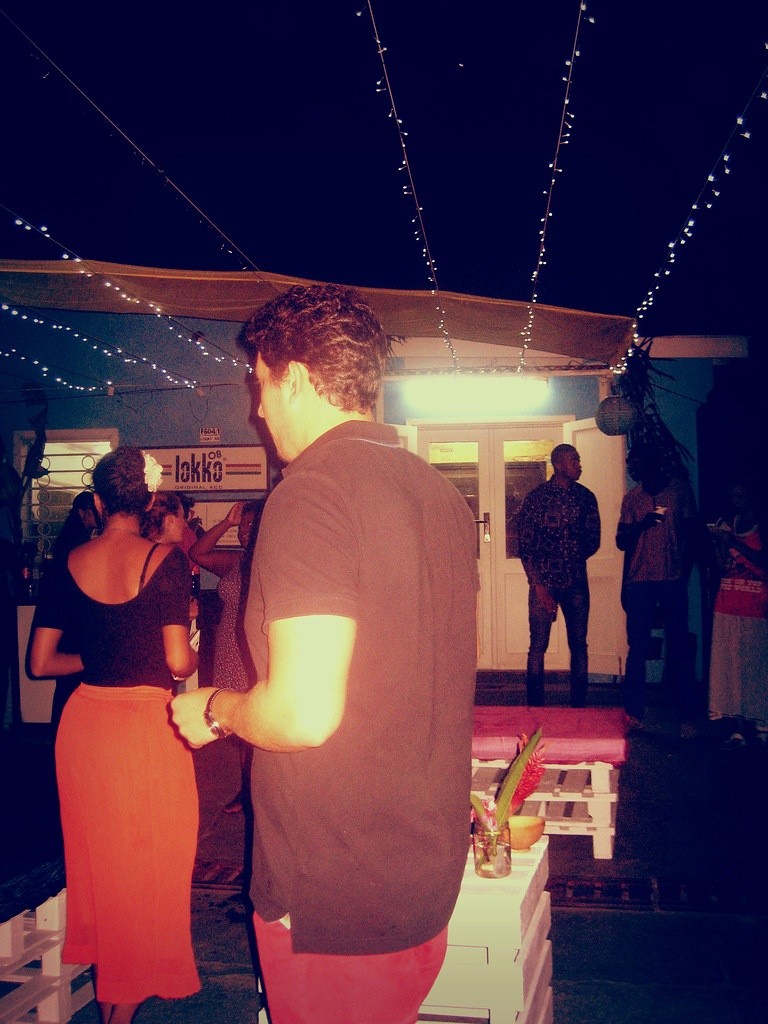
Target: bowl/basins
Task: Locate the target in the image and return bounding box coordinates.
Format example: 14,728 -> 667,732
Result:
508,815 -> 545,852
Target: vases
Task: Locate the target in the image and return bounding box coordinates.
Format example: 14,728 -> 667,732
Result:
474,821 -> 512,880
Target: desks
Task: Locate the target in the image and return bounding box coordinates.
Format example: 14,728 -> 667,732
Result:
256,834 -> 554,1024
467,706 -> 630,862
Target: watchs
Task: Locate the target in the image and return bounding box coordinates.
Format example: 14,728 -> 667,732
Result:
204,688 -> 234,739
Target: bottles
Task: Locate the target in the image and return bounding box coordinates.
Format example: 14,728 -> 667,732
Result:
20,552 -> 31,599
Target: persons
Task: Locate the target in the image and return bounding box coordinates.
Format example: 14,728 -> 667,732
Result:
171,286 -> 478,1024
519,445 -> 601,708
189,501 -> 263,813
31,446 -> 201,1024
145,491 -> 206,690
707,477 -> 768,751
616,448 -> 692,733
51,491 -> 99,747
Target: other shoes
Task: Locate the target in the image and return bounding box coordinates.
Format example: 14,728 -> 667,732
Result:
224,791 -> 243,813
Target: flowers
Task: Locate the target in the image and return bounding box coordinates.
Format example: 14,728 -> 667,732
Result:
469,727 -> 547,870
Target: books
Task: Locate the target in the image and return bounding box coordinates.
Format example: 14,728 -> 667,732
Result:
707,518 -> 732,536
